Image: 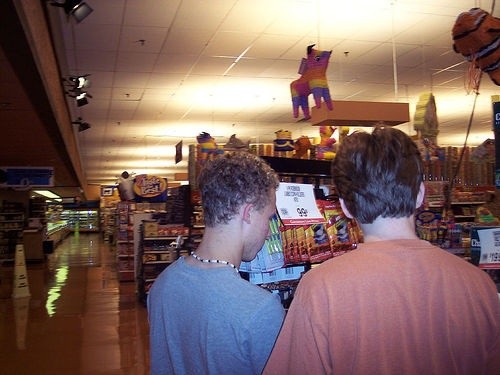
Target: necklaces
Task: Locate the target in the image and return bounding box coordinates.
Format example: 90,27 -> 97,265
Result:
189,250 -> 241,276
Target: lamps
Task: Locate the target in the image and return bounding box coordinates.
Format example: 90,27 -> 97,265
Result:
50,0 -> 94,23
61,74 -> 92,134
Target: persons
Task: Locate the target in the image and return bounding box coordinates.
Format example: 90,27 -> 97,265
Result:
261,122 -> 499,375
145,150 -> 287,375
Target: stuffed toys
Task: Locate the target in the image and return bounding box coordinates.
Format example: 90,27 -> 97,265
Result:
271,46 -> 347,159
195,131 -> 251,162
448,7 -> 500,86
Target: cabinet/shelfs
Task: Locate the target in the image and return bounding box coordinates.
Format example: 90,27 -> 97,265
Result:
0,141 -> 500,311
